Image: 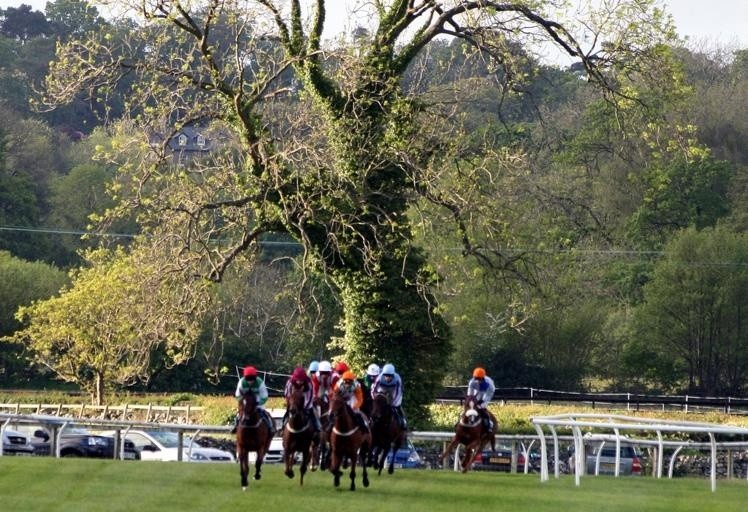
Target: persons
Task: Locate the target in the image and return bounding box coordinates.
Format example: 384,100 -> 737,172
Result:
229,365 -> 277,438
464,367 -> 496,432
281,358 -> 404,455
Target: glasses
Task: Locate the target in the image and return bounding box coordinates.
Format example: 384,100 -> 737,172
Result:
245,375 -> 256,381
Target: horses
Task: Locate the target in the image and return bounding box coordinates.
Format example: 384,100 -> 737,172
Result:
236,388 -> 277,492
282,386 -> 404,490
437,390 -> 499,474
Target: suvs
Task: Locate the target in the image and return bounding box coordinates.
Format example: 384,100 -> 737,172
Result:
566,431 -> 643,476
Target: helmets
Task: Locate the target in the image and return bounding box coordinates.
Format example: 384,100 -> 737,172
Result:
336,362 -> 347,371
318,361 -> 332,372
366,363 -> 379,376
342,371 -> 353,380
473,367 -> 485,378
382,362 -> 395,375
293,368 -> 305,381
243,366 -> 256,377
309,361 -> 318,372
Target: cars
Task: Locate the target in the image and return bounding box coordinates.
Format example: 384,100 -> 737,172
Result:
234,408 -> 423,469
0,413 -> 237,464
472,439 -> 533,473
